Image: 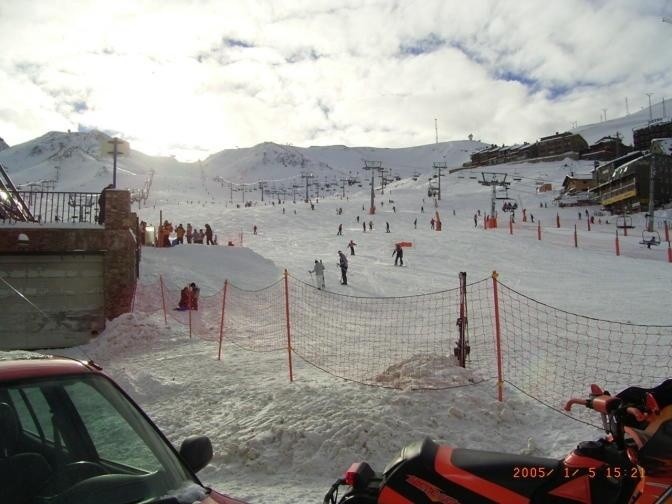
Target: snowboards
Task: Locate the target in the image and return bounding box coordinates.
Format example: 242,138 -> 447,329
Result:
390,263 -> 406,268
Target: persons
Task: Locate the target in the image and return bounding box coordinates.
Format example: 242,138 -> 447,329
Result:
190,282 -> 200,310
191,228 -> 199,244
174,223 -> 186,245
204,223 -> 213,245
337,249 -> 350,285
173,286 -> 190,311
385,221 -> 390,234
97,183 -> 115,225
337,223 -> 343,236
227,240 -> 234,246
391,243 -> 404,267
647,235 -> 656,250
162,219 -> 173,247
346,240 -> 358,256
310,258 -> 326,291
253,224 -> 257,235
184,223 -> 193,244
430,218 -> 437,230
197,228 -> 204,244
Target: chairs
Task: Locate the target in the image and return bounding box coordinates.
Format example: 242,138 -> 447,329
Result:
1,402 -> 53,502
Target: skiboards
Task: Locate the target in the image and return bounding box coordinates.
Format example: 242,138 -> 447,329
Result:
454,271 -> 470,368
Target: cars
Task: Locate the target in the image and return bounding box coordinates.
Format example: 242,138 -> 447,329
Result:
0,350 -> 248,504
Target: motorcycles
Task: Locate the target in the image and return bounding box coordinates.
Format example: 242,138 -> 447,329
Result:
321,379 -> 672,503
